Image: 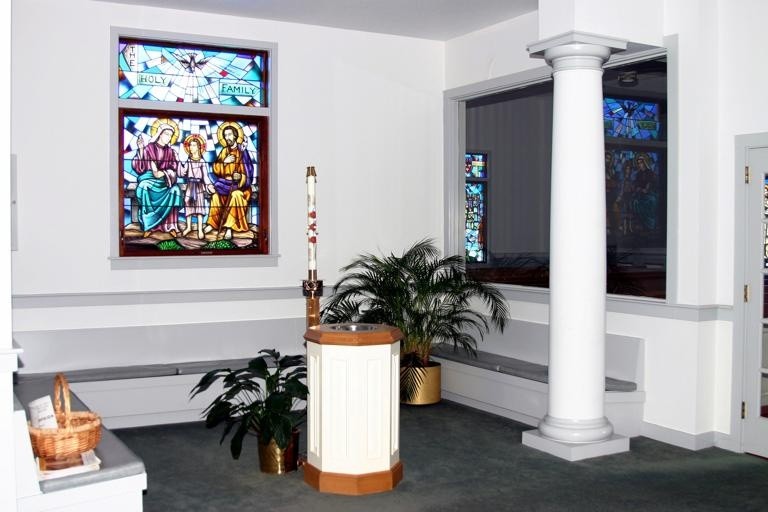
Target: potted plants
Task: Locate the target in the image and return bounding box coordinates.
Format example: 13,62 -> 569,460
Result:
320,234 -> 509,409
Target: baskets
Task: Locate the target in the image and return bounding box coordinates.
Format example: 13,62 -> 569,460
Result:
26,372 -> 104,459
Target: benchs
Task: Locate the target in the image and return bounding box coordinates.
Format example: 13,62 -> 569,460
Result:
13,378 -> 152,512
14,309 -> 429,430
430,310 -> 648,438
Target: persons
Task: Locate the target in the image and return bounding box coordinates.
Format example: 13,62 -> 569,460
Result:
172,138 -> 216,242
604,151 -> 623,238
130,122 -> 181,239
613,162 -> 636,237
631,155 -> 660,235
203,126 -> 255,243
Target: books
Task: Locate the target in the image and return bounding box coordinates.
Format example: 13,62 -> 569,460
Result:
34,449 -> 102,483
